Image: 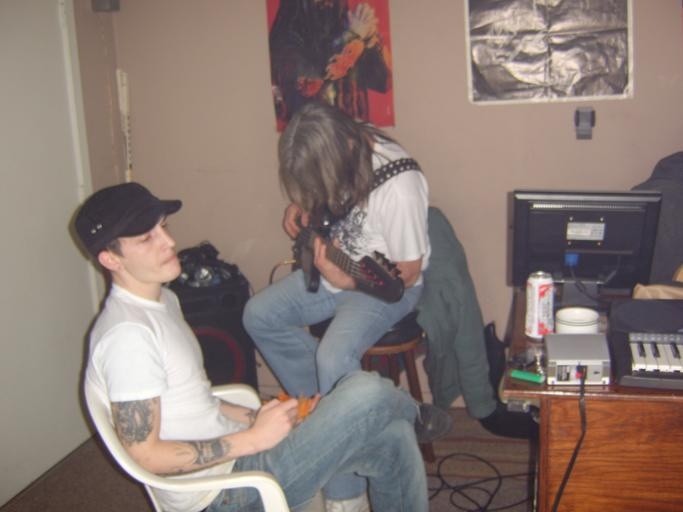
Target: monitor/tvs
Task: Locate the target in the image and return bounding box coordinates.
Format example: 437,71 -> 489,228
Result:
511,189 -> 662,313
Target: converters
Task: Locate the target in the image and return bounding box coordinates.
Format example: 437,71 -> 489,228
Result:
542,333 -> 613,386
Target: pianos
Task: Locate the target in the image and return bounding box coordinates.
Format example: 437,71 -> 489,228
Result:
608,299 -> 683,392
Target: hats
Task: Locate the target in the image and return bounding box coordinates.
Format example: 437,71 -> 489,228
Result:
75,182 -> 183,257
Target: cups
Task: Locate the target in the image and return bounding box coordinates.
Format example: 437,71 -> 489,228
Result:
554,306 -> 600,334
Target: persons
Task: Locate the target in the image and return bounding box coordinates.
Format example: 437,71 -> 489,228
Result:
239,99 -> 432,512
73,180 -> 454,512
268,0 -> 389,130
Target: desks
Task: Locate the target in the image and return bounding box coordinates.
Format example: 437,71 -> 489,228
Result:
500,288 -> 683,512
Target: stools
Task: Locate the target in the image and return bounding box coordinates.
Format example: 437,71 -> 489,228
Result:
359,324 -> 436,464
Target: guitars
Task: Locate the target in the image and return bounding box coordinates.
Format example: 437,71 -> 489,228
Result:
292,200 -> 404,304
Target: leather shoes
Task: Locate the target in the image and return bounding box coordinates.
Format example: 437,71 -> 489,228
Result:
411,402 -> 452,442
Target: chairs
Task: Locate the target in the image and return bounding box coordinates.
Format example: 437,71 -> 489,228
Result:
83,351 -> 294,510
